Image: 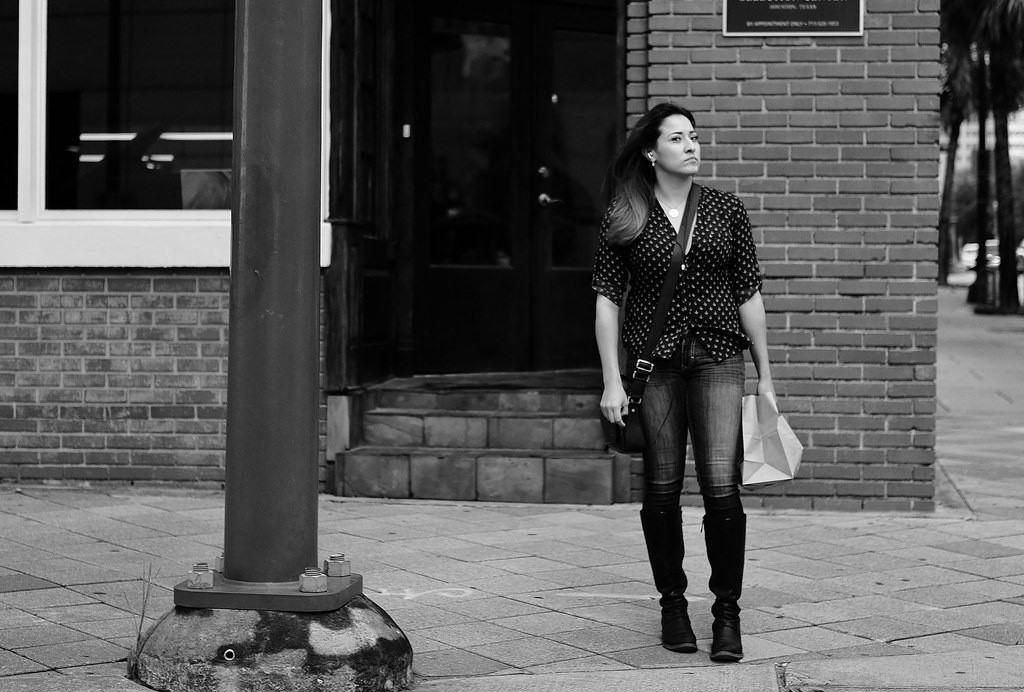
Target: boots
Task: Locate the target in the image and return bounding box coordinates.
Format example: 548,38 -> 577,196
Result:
701,513 -> 746,662
640,507 -> 699,654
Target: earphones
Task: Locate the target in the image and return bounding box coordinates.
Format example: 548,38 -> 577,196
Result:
647,152 -> 653,159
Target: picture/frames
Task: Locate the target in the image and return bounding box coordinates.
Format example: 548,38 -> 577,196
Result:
722,0 -> 864,37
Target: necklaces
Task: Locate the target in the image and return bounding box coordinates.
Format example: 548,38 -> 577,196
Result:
656,196 -> 686,217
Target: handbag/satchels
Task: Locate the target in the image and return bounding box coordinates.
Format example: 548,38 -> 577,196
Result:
738,394 -> 804,486
600,396 -> 648,454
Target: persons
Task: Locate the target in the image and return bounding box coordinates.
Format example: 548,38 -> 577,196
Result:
591,103 -> 777,661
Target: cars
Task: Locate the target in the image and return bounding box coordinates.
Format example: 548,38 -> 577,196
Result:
985,240 -> 1024,273
962,243 -> 979,269
